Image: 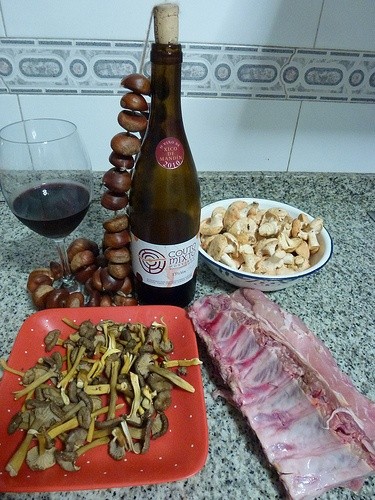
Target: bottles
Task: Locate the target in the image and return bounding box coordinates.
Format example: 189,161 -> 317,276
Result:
127,3 -> 200,308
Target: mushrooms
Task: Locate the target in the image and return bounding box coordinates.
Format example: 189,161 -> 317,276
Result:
199,200 -> 325,275
0,318 -> 205,477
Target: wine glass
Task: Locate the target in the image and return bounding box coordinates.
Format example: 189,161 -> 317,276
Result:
0,118 -> 93,304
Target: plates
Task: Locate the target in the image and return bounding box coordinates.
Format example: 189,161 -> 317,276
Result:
0,306 -> 208,492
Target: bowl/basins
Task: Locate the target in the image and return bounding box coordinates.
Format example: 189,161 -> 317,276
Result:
198,197 -> 334,291
